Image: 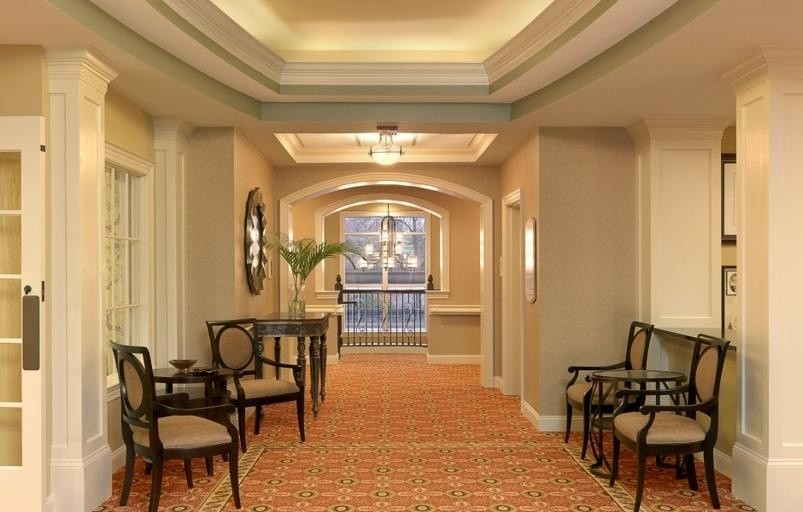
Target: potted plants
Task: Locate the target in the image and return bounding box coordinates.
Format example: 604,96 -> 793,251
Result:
258,229 -> 368,315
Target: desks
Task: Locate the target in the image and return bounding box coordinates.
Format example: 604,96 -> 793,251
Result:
589,368 -> 688,480
144,367 -> 234,476
252,311 -> 330,416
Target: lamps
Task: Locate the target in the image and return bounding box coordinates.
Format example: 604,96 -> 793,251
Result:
368,124 -> 406,166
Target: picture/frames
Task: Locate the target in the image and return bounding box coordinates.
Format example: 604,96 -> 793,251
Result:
267,255 -> 273,280
721,152 -> 737,243
721,265 -> 738,350
524,215 -> 537,305
244,186 -> 267,296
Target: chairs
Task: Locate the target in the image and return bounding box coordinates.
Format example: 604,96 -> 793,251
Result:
564,321 -> 655,460
609,330 -> 731,512
108,339 -> 241,512
206,317 -> 306,453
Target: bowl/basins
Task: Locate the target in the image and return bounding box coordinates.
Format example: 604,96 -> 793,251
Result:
169,359 -> 197,374
